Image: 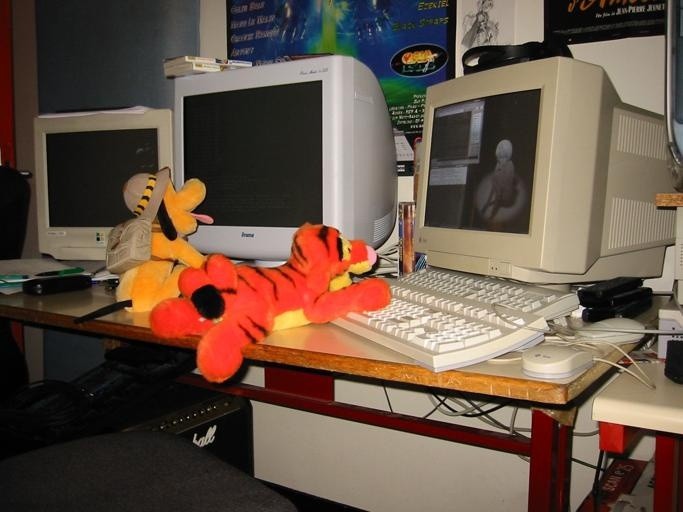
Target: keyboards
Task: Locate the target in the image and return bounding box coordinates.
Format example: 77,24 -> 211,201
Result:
321,268 -> 581,378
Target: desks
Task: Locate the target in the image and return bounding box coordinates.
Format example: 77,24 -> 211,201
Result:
0,259 -> 675,512
591,341 -> 683,511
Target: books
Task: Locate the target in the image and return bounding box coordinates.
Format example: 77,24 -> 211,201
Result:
164,55 -> 253,80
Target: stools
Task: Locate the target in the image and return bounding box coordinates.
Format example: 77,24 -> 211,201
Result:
0,429 -> 300,512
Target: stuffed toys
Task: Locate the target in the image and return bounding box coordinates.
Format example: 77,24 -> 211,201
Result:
148,221 -> 392,382
116,167 -> 214,312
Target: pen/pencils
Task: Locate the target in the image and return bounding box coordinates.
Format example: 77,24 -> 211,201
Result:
92,281 -> 100,284
35,267 -> 85,276
0,275 -> 29,287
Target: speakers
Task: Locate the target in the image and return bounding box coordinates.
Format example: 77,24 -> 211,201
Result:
80,386 -> 255,480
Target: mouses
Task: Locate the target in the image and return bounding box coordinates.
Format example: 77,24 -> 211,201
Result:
585,318 -> 647,350
524,346 -> 591,386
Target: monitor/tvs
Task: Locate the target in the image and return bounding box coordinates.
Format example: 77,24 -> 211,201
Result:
412,55 -> 677,288
33,109 -> 171,261
174,54 -> 398,273
